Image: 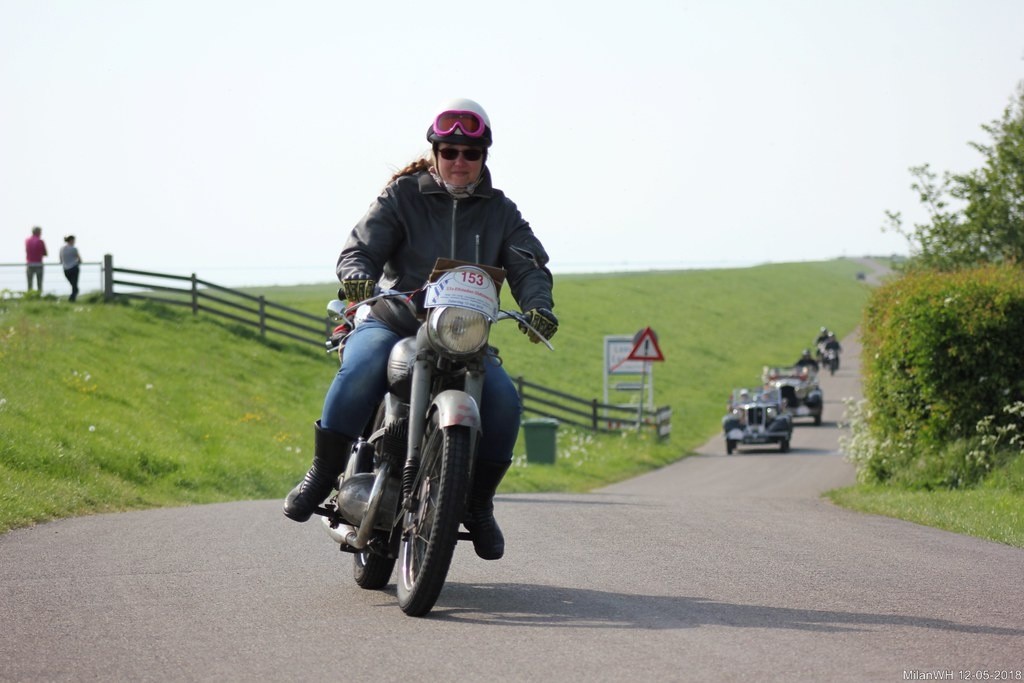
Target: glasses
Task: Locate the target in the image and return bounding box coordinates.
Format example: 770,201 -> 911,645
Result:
435,148 -> 485,161
433,111 -> 486,137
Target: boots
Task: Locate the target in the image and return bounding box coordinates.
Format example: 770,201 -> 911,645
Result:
462,457 -> 511,560
282,420 -> 355,520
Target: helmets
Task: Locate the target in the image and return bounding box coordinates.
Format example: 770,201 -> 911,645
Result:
427,99 -> 491,145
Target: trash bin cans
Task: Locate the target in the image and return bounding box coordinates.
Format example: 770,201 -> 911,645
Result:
523,418 -> 559,465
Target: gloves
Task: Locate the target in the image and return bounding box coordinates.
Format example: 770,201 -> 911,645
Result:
517,307 -> 559,343
342,269 -> 375,304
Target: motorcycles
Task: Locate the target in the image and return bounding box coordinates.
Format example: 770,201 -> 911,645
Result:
815,342 -> 841,375
724,384 -> 795,455
762,365 -> 825,426
315,265 -> 554,619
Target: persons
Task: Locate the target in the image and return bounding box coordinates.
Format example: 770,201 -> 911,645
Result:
741,327 -> 843,403
59,236 -> 82,303
24,227 -> 48,295
282,98 -> 557,560
